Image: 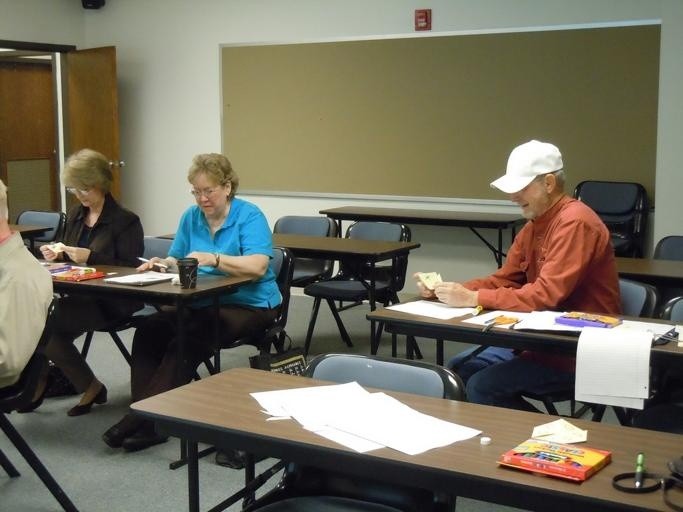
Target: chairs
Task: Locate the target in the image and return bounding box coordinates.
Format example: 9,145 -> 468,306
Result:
301,221 -> 423,358
659,297 -> 683,325
652,234 -> 682,262
565,180 -> 650,256
254,351 -> 458,512
202,246 -> 294,376
0,415 -> 80,511
514,280 -> 657,419
15,209 -> 65,248
269,213 -> 339,351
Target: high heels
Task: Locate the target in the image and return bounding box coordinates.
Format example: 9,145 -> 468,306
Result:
67,383 -> 108,417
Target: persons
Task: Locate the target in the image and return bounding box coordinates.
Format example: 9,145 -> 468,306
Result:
411,138 -> 622,413
101,152 -> 281,452
16,146 -> 145,417
0,178 -> 55,398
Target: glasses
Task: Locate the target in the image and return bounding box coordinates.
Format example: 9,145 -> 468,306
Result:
66,187 -> 96,195
192,187 -> 222,198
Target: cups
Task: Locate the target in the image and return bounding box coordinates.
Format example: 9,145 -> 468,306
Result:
176,258 -> 198,288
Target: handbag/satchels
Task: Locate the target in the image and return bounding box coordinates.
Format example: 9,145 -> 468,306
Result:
248,327 -> 308,377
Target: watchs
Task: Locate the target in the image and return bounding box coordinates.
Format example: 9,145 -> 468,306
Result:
212,252 -> 221,268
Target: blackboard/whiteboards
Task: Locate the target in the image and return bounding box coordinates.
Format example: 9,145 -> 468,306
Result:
219,19 -> 661,213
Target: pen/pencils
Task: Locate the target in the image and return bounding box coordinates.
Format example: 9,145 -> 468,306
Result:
485,315 -> 504,324
136,257 -> 168,269
635,453 -> 644,488
515,452 -> 582,469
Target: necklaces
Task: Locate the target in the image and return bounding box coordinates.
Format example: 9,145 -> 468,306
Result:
208,209 -> 224,226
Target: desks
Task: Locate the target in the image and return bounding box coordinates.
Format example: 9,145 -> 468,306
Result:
36,260 -> 260,310
367,296 -> 683,366
128,366 -> 682,510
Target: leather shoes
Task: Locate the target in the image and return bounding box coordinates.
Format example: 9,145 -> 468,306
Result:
102,414 -> 166,451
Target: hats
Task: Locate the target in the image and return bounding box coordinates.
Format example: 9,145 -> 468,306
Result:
489,140 -> 564,194
